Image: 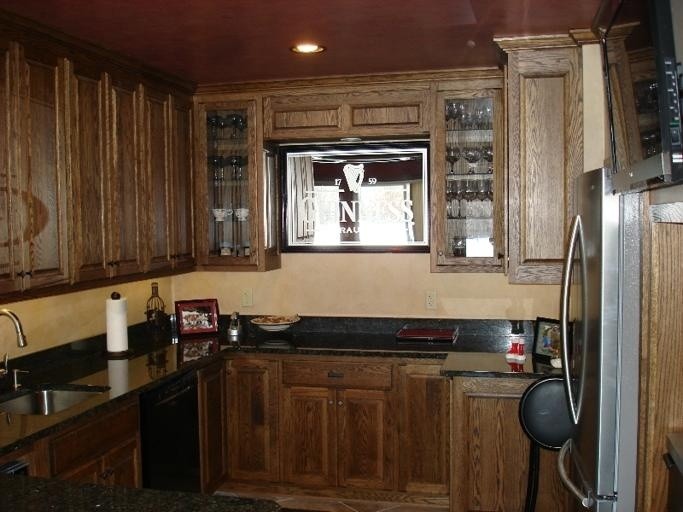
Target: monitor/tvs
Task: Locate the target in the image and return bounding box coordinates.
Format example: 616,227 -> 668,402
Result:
589,0 -> 682,195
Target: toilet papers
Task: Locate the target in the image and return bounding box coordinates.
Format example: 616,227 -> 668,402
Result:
106,298 -> 128,352
107,359 -> 129,399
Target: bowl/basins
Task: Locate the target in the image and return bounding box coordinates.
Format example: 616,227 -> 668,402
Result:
257,339 -> 295,353
250,315 -> 301,331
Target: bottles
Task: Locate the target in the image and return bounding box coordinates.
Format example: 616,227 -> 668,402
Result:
217,240 -> 251,257
507,359 -> 525,373
445,240 -> 463,257
507,338 -> 526,356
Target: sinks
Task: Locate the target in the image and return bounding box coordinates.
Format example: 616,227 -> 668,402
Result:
0,383 -> 112,415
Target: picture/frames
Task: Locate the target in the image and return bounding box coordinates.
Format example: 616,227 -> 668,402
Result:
175,299 -> 219,340
532,317 -> 573,366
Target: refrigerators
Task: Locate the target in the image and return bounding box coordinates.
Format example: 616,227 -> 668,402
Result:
557,166 -> 639,510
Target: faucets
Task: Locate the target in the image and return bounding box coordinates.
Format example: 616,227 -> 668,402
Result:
0,309 -> 27,347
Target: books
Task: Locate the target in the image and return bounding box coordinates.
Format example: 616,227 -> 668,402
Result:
396,322 -> 459,340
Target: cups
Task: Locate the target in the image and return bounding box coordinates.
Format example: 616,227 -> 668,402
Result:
211,207 -> 252,224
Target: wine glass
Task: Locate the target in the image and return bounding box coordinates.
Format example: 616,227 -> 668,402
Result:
446,100 -> 496,237
208,113 -> 246,183
